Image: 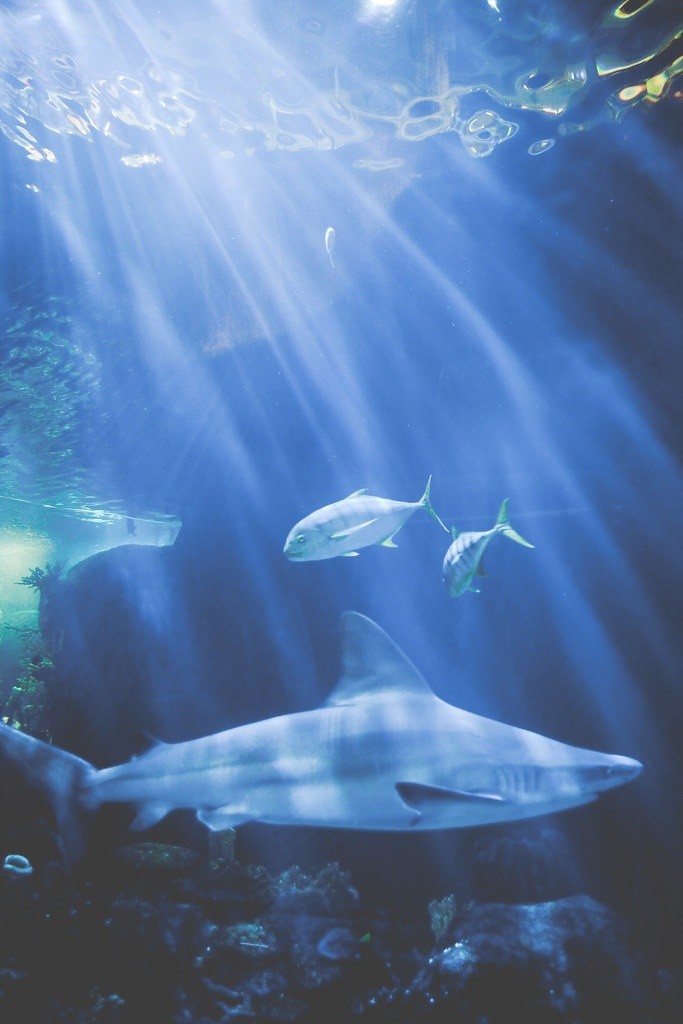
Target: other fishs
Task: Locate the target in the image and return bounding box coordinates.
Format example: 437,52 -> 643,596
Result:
441,496 -> 539,600
325,226 -> 336,267
283,474 -> 449,564
